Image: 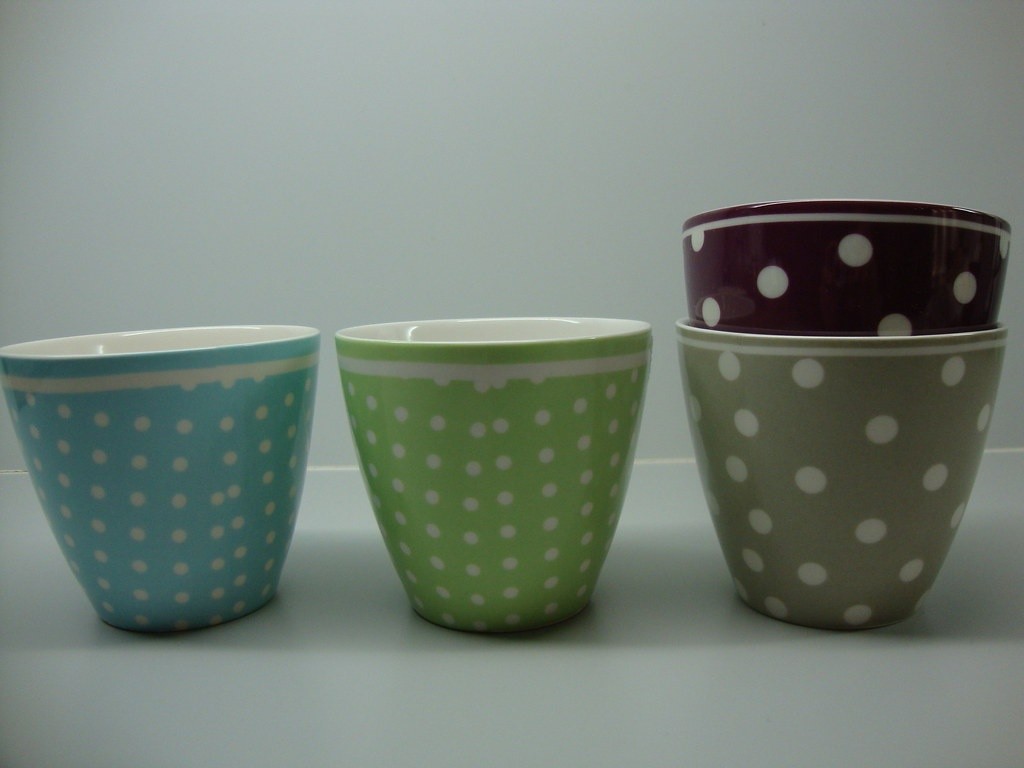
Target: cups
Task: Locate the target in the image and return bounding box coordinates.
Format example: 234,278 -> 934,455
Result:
334,317 -> 653,635
676,318 -> 1008,630
0,325 -> 319,634
681,198 -> 1012,337
